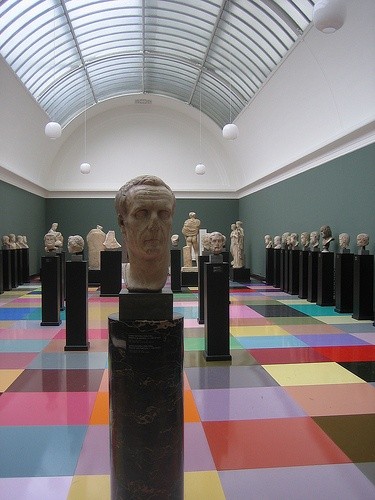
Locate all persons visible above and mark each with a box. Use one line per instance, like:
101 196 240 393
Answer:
338 232 350 249
200 233 212 251
357 233 369 247
232 220 244 269
182 212 200 267
310 231 320 247
43 223 64 252
0 233 30 249
301 232 310 247
208 232 227 252
67 235 84 254
114 175 174 292
320 225 335 246
229 224 238 266
264 231 299 250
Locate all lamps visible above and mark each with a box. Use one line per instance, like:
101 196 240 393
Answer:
313 0 345 34
222 50 240 140
45 0 62 140
80 65 91 174
196 75 206 175
